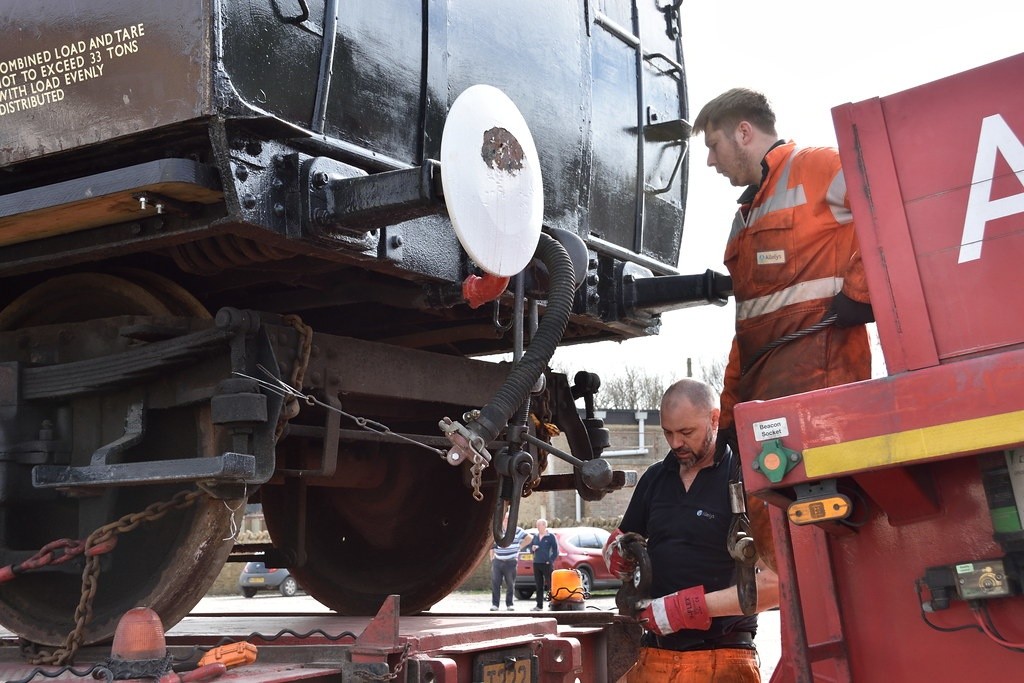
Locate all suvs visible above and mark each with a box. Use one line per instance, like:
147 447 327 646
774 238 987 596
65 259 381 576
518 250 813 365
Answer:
512 526 625 603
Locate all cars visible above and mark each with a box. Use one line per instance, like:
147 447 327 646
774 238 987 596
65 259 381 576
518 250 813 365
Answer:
239 548 304 599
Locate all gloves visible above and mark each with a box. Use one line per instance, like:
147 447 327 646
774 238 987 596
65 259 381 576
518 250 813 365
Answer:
714 426 741 467
600 528 649 582
822 291 875 325
632 585 712 636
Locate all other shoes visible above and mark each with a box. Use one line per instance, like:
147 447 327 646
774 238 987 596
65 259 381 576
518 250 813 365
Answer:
530 606 544 611
490 605 499 611
506 605 514 611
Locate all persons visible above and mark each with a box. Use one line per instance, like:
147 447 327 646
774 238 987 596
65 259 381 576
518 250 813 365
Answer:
601 378 780 683
691 88 876 574
490 512 559 611
